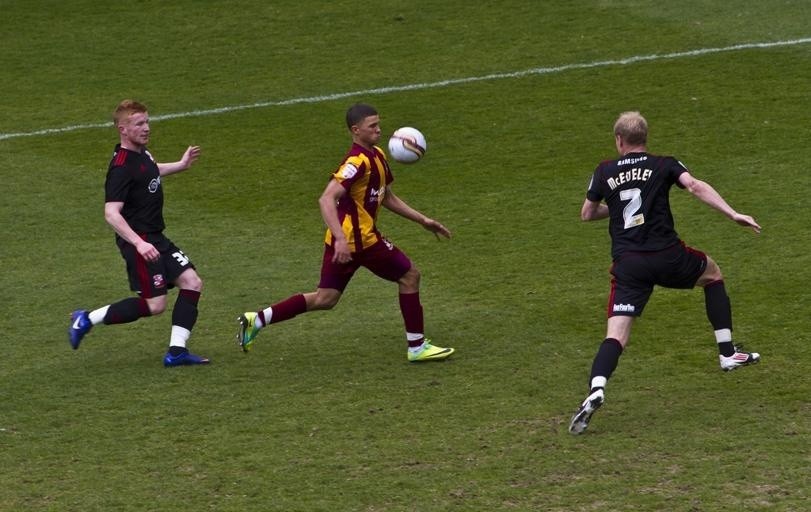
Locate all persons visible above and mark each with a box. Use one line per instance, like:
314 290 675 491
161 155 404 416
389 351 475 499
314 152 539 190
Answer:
69 98 210 367
235 103 455 363
569 111 762 438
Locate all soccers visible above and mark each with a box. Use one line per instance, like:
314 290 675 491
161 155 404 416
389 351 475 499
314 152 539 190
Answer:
388 127 425 164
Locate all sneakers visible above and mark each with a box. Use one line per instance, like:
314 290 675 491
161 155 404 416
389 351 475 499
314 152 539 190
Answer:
569 387 604 435
407 338 455 362
164 349 211 367
236 312 260 353
719 346 761 373
68 309 92 350
